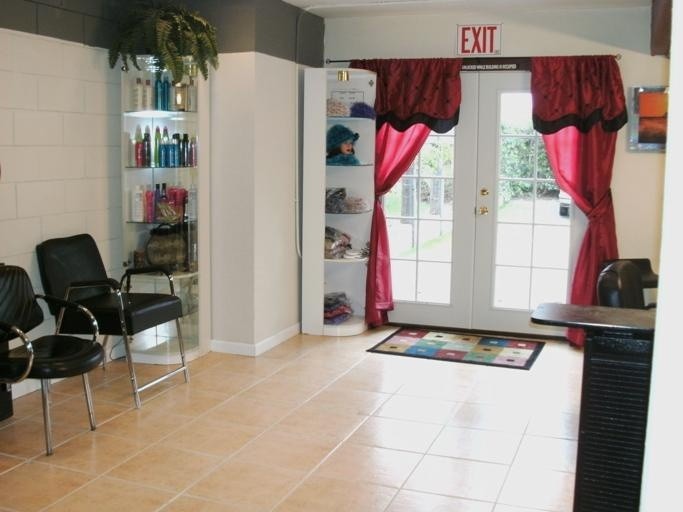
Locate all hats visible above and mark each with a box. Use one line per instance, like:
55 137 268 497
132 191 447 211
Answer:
326 188 370 213
326 126 358 149
325 99 349 118
351 102 376 117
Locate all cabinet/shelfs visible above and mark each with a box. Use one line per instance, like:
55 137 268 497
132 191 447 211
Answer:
302 67 376 336
121 55 212 365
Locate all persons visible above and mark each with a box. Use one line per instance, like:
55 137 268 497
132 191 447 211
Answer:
326 123 360 157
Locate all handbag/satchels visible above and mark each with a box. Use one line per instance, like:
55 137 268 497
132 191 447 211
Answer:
145 224 186 265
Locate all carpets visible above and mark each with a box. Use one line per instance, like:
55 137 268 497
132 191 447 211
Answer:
366 327 546 371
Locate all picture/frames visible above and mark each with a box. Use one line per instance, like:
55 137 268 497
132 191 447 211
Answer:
627 85 670 152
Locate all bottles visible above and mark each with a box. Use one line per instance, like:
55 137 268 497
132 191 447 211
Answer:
131 182 196 222
129 124 197 168
132 74 197 111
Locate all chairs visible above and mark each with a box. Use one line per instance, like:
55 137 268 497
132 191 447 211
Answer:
596 259 658 311
0 265 105 456
36 231 189 407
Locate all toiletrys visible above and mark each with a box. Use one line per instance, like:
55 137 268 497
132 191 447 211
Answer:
159 137 180 168
135 76 169 111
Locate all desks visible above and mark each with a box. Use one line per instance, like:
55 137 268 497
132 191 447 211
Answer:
531 302 655 512
